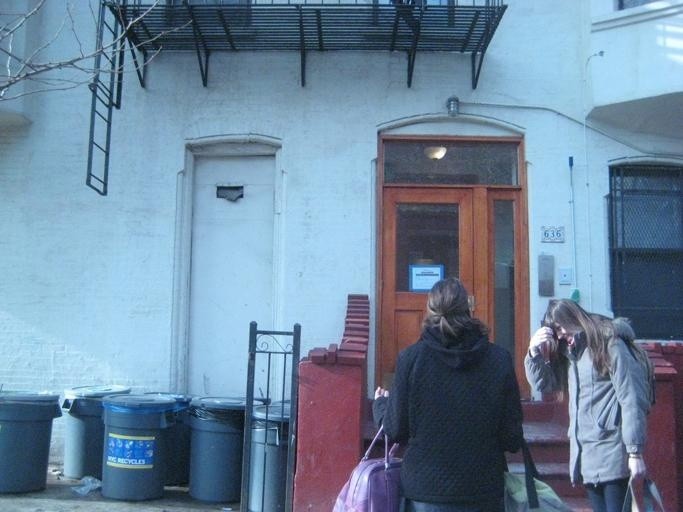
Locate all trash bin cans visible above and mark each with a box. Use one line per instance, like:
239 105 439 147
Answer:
184 396 270 504
165 392 191 487
62 385 131 479
1 391 62 493
101 394 178 501
247 405 290 512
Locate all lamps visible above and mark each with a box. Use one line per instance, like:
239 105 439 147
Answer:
446 94 460 111
422 145 447 162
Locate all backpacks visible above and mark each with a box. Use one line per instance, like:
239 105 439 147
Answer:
622 335 655 415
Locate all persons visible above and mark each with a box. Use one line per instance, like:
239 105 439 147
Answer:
372 279 523 512
524 299 656 512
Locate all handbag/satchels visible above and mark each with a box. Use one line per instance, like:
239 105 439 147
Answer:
331 457 403 512
504 475 573 512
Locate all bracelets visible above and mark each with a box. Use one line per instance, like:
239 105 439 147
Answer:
629 456 641 459
